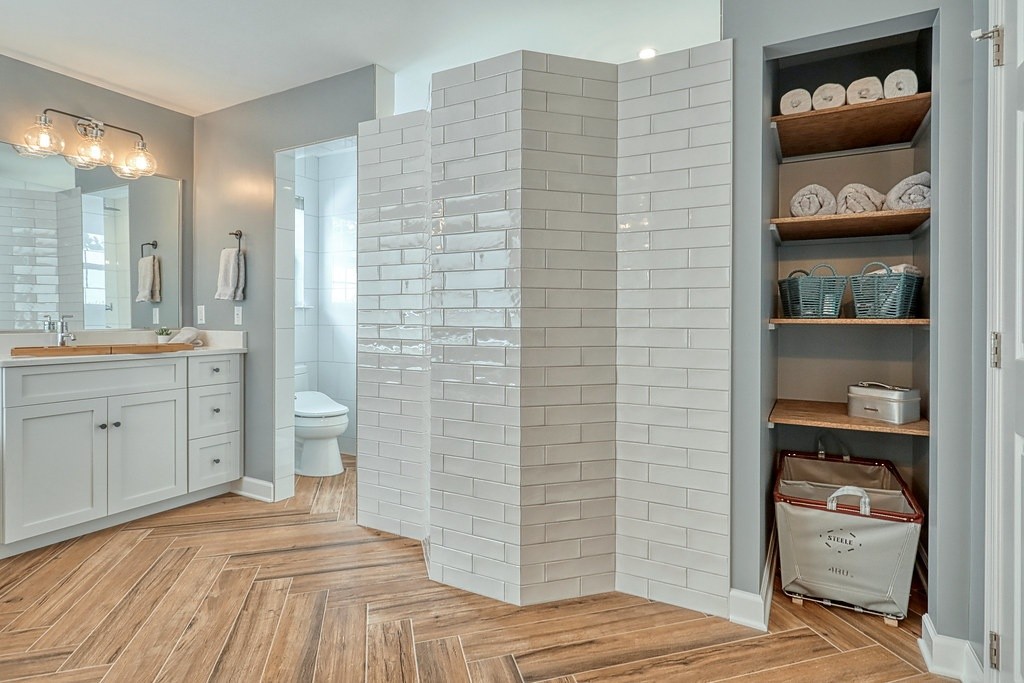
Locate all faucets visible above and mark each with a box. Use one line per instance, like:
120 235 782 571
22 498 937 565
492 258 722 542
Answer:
43 315 55 333
57 315 77 347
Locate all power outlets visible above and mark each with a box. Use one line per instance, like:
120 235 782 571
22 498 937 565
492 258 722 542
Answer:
153 308 159 325
234 306 243 326
197 305 205 325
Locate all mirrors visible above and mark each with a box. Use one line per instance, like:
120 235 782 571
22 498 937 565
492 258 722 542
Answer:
0 141 184 335
294 195 304 309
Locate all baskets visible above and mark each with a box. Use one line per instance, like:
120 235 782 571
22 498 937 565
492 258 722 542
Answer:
777 264 849 318
850 262 924 318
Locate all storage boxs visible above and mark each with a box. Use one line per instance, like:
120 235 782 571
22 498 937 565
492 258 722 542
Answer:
773 448 925 628
847 381 921 425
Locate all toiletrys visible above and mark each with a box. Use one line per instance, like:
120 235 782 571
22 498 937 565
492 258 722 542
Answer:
822 280 838 316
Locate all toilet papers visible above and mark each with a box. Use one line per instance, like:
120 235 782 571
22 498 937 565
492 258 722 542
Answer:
780 69 919 116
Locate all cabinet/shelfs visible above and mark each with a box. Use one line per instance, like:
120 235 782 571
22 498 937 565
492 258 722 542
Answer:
0 353 245 559
767 90 932 444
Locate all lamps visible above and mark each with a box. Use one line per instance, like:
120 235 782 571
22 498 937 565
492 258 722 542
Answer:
12 144 142 180
25 108 158 177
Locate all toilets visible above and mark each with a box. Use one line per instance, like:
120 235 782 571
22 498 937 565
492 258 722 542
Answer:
294 365 350 477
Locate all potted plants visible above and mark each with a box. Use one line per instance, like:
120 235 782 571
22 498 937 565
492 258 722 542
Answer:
154 327 172 345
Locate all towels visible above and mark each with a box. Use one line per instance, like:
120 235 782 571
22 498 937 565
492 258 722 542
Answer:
135 255 161 305
213 249 246 302
168 327 203 347
789 171 932 217
856 263 922 317
